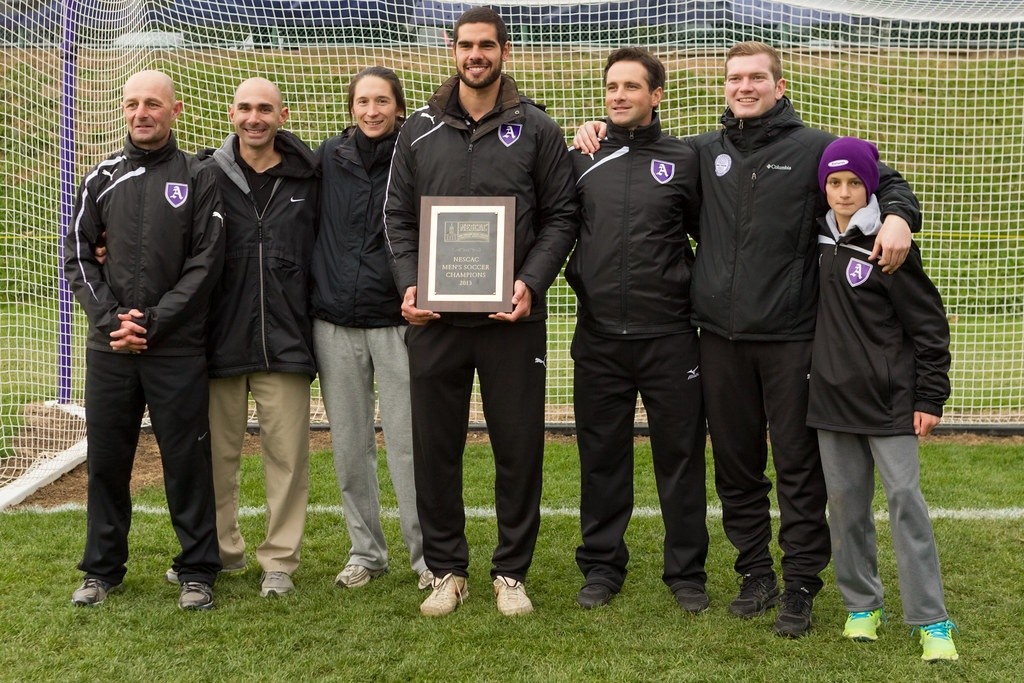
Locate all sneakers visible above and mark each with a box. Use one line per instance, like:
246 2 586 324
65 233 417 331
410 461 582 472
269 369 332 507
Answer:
728 569 777 617
673 586 710 613
177 582 214 611
166 565 251 584
842 609 883 641
492 575 533 617
261 572 295 597
421 575 470 617
419 569 434 590
335 565 385 589
579 585 613 609
919 621 961 663
72 579 123 607
773 590 815 640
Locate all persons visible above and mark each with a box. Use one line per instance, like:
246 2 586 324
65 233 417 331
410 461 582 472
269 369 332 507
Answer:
314 66 436 590
93 77 322 597
806 138 959 662
381 8 579 617
572 42 925 639
63 69 225 613
556 47 708 610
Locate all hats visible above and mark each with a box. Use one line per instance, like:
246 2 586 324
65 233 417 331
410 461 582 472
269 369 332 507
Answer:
817 137 880 202
